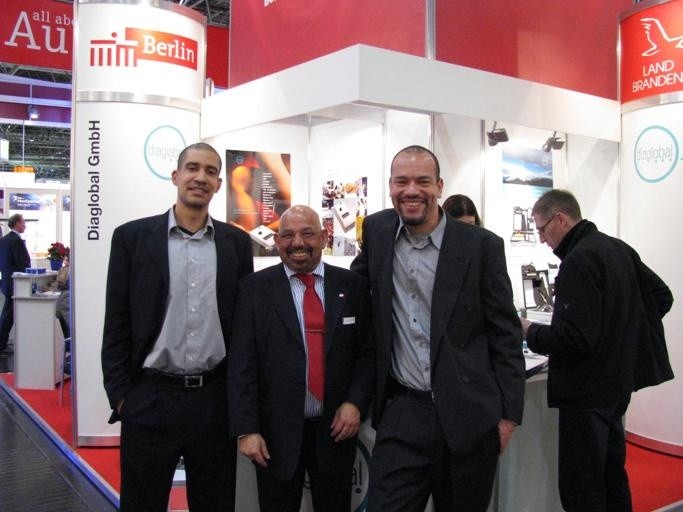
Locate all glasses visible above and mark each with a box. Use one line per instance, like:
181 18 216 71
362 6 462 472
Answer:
535 212 559 234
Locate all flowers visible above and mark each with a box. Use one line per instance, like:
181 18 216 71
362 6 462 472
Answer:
48 242 65 261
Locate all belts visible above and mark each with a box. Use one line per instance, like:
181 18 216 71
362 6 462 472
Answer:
142 366 228 389
392 379 435 403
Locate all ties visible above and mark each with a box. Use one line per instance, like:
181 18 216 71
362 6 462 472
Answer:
295 273 328 401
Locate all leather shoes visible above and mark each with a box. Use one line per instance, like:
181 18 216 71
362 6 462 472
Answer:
0 347 14 355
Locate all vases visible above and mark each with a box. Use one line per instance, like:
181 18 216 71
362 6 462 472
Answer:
49 255 62 270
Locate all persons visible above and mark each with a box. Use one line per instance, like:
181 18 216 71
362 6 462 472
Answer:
439 194 482 229
512 189 678 509
0 213 32 357
222 203 380 511
38 252 71 366
98 140 255 512
227 151 293 237
345 143 526 511
